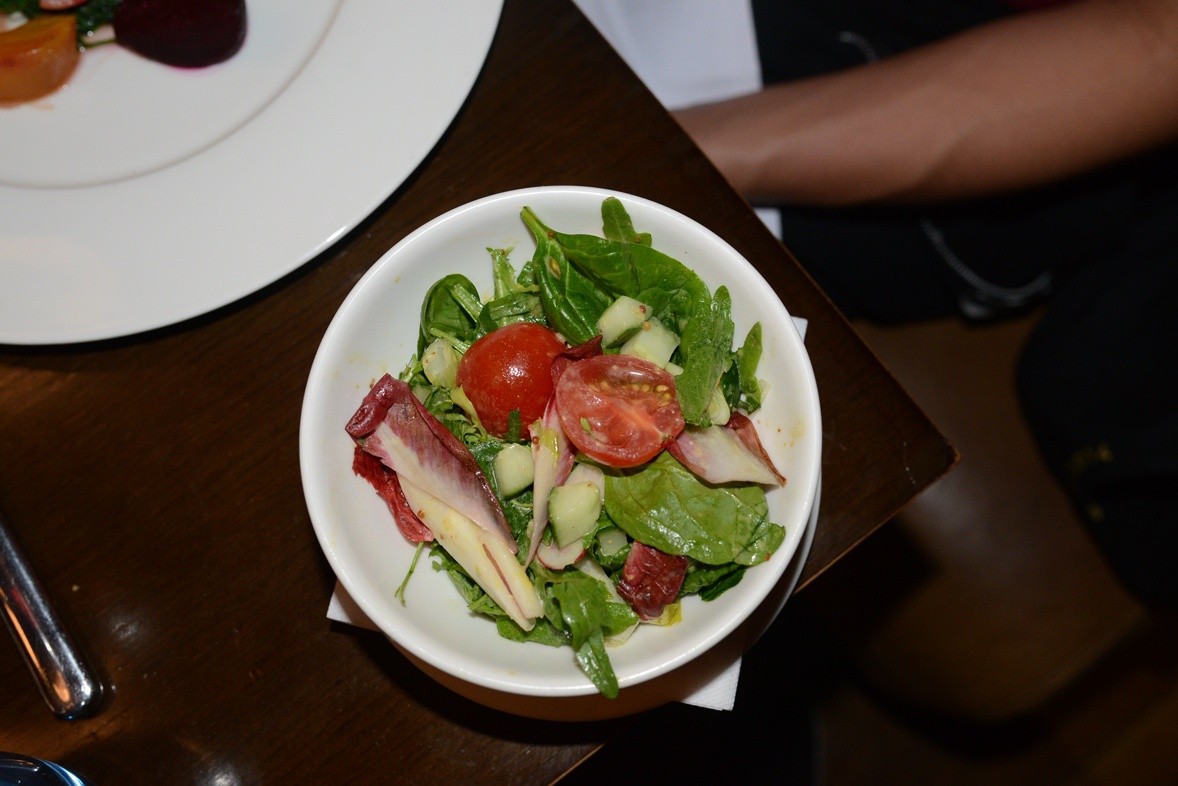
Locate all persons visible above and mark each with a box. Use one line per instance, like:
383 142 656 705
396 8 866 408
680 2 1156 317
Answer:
667 1 1178 306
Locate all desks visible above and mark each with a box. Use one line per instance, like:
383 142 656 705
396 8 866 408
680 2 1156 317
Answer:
0 0 963 786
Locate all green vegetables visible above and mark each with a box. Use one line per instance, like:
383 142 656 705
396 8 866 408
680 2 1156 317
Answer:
0 0 118 47
341 197 785 701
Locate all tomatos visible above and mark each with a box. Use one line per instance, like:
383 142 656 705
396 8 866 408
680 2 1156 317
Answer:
455 321 569 442
554 354 687 467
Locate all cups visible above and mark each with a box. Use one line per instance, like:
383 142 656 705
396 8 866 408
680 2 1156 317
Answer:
0 749 86 786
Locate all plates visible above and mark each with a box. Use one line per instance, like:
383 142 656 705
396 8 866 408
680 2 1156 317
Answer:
296 184 825 697
0 0 506 349
388 468 820 724
